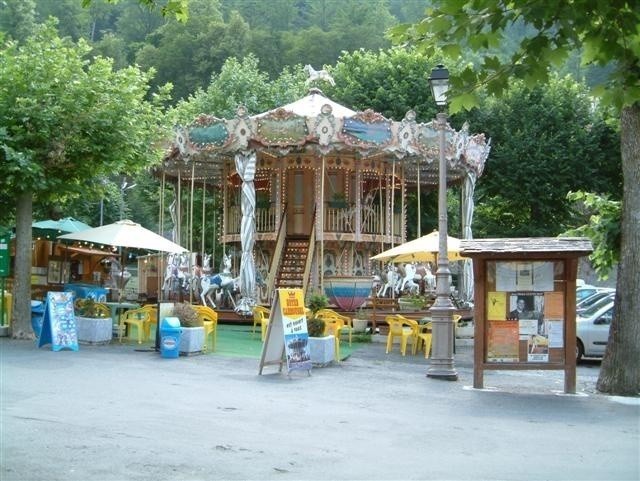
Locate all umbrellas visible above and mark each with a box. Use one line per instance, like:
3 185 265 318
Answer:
368 229 471 290
55 219 190 325
12 217 94 284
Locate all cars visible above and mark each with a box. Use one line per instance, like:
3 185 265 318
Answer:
576 279 618 362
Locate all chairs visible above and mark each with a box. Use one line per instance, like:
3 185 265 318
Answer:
384 314 461 360
252 307 351 360
119 304 217 355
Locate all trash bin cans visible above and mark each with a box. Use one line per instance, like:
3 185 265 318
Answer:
30 300 45 336
160 317 182 358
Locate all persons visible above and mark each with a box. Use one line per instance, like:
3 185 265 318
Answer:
508 297 532 320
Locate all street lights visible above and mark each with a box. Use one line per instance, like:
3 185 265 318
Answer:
119 181 137 220
425 62 461 381
